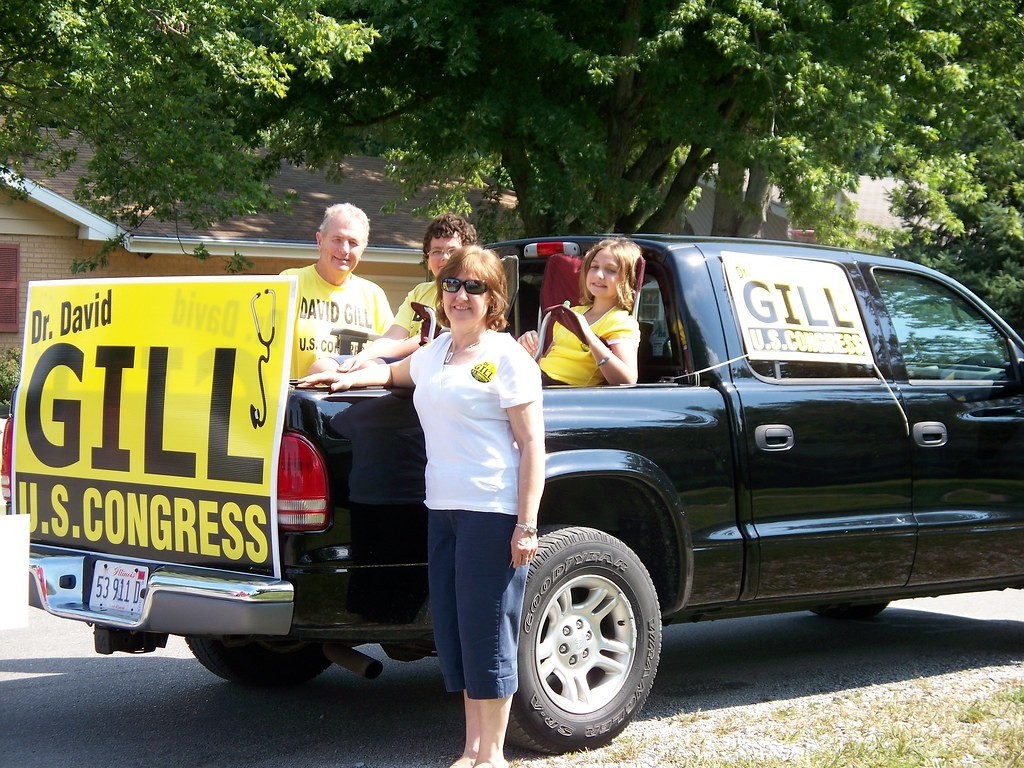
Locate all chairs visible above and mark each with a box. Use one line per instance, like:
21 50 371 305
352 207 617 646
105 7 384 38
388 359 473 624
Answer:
331 255 521 361
538 252 645 328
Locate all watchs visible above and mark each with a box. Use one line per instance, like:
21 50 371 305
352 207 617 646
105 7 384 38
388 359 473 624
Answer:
516 522 537 533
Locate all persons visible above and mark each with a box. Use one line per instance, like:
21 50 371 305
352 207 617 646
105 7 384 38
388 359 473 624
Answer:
516 234 645 388
295 246 546 768
282 201 395 378
344 211 478 389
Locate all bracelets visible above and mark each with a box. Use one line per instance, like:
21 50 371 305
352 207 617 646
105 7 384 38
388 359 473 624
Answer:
596 350 613 365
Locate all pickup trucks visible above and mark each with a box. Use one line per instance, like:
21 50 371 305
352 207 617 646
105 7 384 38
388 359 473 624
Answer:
2 231 1022 757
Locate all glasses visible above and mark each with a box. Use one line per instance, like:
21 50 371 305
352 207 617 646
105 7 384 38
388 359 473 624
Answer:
441 278 487 294
427 248 455 256
